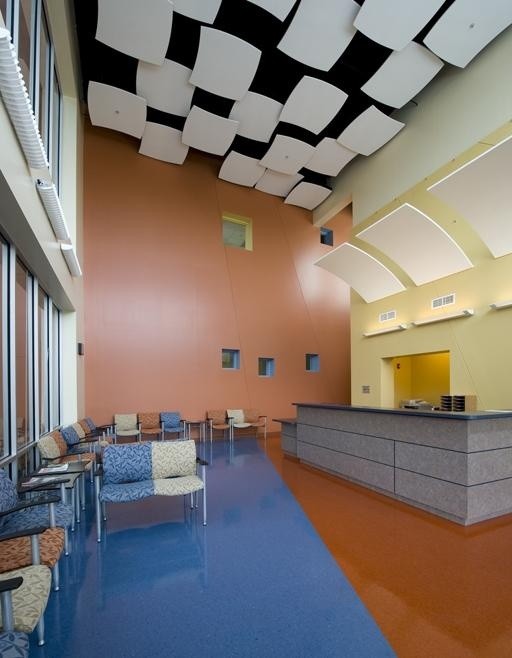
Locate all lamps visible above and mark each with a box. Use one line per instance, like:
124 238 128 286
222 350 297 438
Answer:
62 243 82 277
490 300 512 310
0 28 49 169
363 324 407 336
412 309 473 327
36 180 70 240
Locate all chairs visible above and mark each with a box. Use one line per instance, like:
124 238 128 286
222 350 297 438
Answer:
1 411 111 656
96 440 208 533
114 408 266 442
